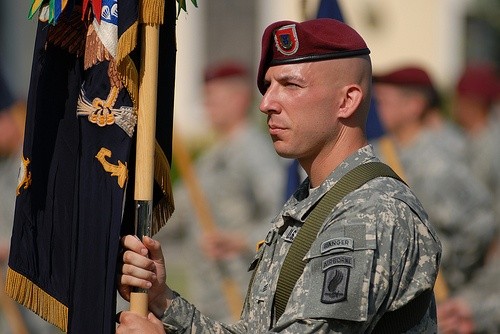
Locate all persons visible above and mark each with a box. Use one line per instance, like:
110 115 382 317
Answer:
153 58 291 322
0 75 61 334
371 58 500 334
115 18 442 334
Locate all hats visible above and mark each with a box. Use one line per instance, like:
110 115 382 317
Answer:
457 65 500 99
204 63 250 81
373 68 431 85
257 19 371 96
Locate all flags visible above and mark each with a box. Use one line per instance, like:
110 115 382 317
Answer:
282 0 386 208
6 0 191 332
